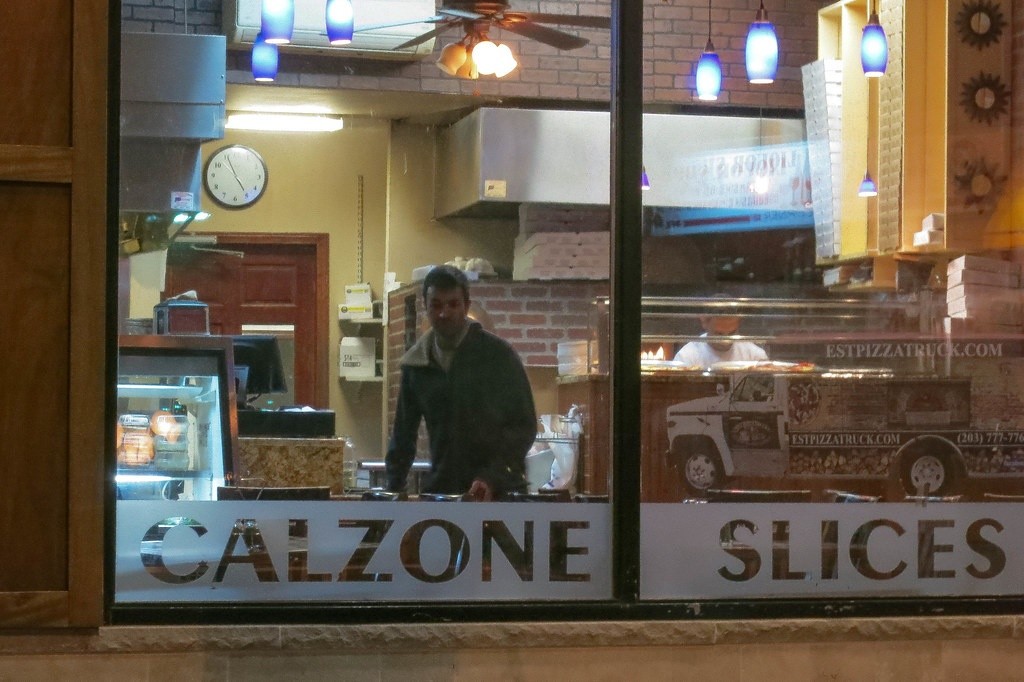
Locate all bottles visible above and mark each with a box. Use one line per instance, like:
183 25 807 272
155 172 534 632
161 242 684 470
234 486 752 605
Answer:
342 435 357 488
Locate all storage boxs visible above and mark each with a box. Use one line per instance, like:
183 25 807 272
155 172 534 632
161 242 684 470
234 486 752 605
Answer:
946 254 1020 319
338 336 376 378
338 284 373 319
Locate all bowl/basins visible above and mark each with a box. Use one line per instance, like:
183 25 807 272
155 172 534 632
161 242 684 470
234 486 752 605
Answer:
124 317 153 334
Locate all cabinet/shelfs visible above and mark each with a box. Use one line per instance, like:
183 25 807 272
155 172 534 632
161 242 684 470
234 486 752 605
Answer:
347 317 383 402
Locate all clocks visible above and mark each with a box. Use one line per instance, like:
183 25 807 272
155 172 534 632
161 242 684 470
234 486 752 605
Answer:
204 145 268 209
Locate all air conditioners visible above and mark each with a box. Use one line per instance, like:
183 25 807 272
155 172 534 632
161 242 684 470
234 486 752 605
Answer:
221 0 436 63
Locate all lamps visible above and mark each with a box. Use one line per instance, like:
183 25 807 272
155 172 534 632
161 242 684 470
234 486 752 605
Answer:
861 0 888 78
325 0 353 45
261 0 295 44
251 32 281 82
744 0 780 84
858 137 878 196
693 0 722 101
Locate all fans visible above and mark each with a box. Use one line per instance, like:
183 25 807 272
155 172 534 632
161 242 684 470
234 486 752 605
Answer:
353 0 611 79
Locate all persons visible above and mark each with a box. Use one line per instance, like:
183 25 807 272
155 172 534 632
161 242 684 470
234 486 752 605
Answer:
385 265 537 501
673 294 768 361
